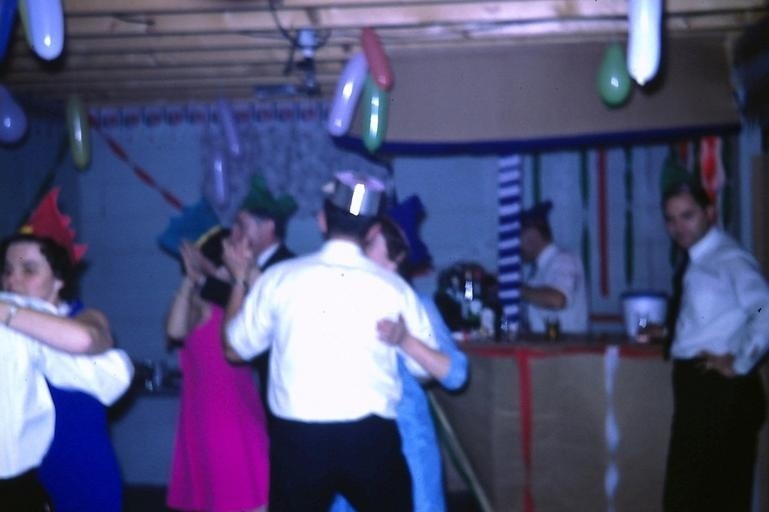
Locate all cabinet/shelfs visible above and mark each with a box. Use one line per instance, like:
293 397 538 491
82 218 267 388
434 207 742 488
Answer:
422 329 674 511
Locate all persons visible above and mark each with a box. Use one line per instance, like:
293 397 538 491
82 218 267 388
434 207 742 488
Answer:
222 168 440 511
1 292 136 509
329 192 471 512
636 162 769 510
1 187 124 510
488 199 589 337
176 184 303 305
165 196 272 512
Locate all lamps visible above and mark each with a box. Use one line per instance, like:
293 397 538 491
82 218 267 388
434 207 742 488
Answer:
276 26 323 100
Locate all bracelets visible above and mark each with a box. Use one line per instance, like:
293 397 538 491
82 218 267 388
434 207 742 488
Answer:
661 325 668 339
178 287 193 304
232 277 250 291
3 303 20 328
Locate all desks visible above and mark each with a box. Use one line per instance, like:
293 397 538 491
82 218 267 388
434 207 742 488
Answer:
108 375 183 487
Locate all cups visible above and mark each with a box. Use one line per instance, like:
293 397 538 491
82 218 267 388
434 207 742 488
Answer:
545 316 558 341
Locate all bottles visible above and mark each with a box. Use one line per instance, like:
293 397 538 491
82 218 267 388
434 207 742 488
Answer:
451 265 486 330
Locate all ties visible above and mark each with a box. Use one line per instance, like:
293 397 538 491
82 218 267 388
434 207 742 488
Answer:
662 249 688 360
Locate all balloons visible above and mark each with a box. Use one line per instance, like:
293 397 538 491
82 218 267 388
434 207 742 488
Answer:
324 53 363 136
1 1 72 61
1 84 29 144
363 76 389 151
212 148 229 206
627 0 663 84
65 92 93 169
363 27 392 86
596 43 629 104
221 99 244 158
